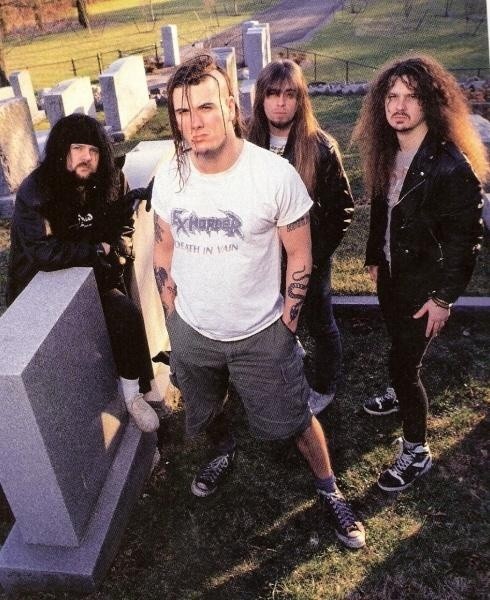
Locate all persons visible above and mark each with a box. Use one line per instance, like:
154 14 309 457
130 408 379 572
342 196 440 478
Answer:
245 57 356 417
4 114 163 434
150 55 367 551
342 53 488 493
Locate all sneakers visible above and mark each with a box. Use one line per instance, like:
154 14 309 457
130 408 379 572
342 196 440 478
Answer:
192 453 231 496
126 394 159 433
363 389 399 414
309 388 334 416
318 488 365 549
377 441 433 490
